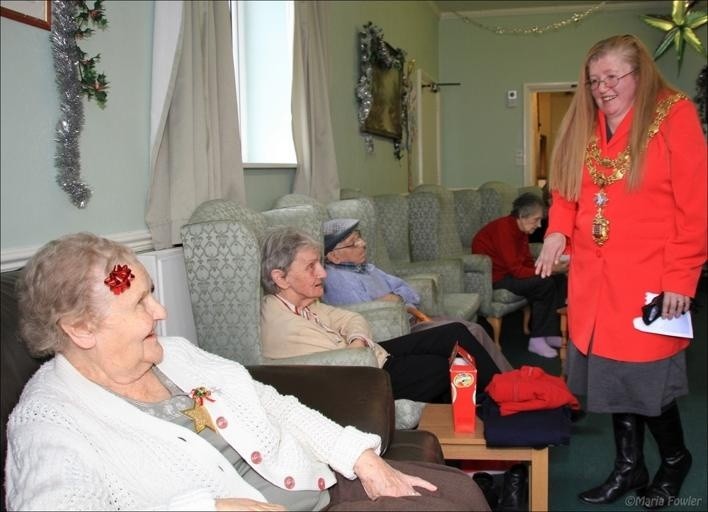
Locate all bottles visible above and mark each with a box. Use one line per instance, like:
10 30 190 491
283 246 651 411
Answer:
323 218 361 256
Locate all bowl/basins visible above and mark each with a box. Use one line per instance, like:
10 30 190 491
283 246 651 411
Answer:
577 94 692 245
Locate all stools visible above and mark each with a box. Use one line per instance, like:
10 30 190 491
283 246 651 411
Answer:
414 402 549 511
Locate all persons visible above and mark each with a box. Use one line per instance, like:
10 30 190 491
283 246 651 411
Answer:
528 33 707 510
257 226 506 410
311 218 520 378
465 192 570 361
0 230 498 512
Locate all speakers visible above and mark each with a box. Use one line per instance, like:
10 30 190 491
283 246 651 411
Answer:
577 411 650 506
643 397 694 511
472 463 529 511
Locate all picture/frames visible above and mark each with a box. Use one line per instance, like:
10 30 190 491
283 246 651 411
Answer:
362 25 406 140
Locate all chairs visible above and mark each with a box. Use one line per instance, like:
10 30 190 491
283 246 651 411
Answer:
1 179 544 512
528 336 562 359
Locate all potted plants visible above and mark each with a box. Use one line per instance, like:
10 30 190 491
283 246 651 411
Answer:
362 25 406 140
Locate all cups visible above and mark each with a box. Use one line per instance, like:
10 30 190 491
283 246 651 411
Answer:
333 230 360 251
584 67 640 91
